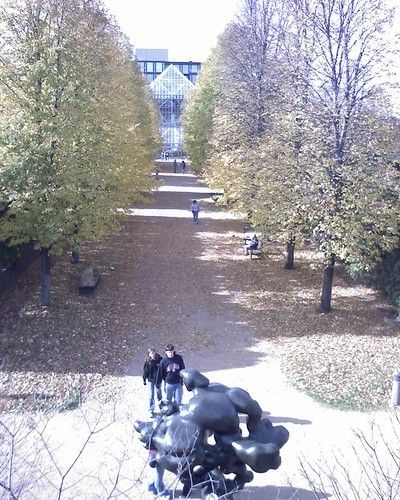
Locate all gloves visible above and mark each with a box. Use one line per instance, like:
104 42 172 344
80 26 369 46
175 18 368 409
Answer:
143 382 146 386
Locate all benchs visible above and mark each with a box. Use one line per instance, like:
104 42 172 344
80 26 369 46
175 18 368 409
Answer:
243 238 263 259
211 192 229 205
79 268 101 297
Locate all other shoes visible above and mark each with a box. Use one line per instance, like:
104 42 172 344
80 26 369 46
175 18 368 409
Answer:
150 412 154 418
160 407 164 413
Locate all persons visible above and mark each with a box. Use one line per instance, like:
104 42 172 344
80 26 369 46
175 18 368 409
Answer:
180 160 185 174
157 344 185 408
143 348 164 419
244 233 259 255
173 159 177 173
192 200 199 224
